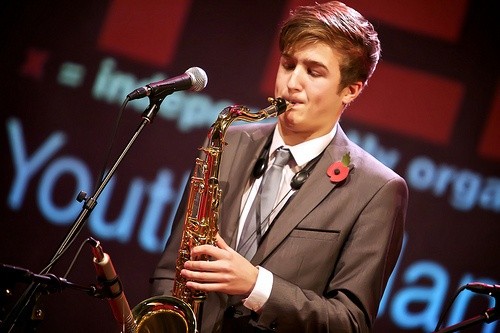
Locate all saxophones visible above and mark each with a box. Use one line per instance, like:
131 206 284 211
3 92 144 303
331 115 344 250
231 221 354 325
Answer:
122 96 294 333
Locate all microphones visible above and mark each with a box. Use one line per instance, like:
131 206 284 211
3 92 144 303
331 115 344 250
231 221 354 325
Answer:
466 282 500 298
127 67 208 101
91 241 138 333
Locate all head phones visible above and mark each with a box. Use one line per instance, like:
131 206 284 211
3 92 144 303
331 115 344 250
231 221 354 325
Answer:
252 127 330 190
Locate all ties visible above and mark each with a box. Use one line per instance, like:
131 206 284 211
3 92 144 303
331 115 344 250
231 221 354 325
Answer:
235 145 291 262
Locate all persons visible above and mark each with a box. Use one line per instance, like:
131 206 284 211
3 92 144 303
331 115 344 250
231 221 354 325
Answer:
151 0 409 333
